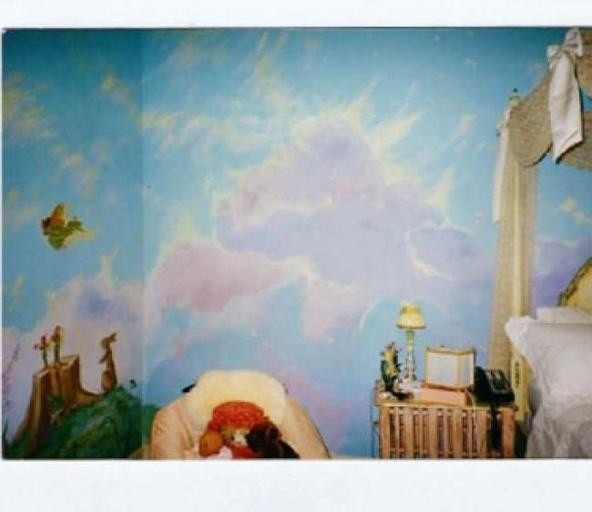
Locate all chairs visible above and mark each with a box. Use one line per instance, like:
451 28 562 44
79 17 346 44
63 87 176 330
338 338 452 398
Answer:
151 368 333 459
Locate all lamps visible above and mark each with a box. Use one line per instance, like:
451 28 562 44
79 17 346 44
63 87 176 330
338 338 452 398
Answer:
395 305 427 381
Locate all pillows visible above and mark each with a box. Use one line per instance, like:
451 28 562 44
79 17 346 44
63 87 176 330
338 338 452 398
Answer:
504 306 592 422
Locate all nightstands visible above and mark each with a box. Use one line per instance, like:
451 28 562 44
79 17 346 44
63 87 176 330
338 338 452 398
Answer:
375 379 519 458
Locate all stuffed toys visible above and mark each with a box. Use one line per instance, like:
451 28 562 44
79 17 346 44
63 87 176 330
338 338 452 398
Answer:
198 400 301 459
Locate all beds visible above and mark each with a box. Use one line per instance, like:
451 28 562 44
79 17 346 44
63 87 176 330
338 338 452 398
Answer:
489 29 592 458
